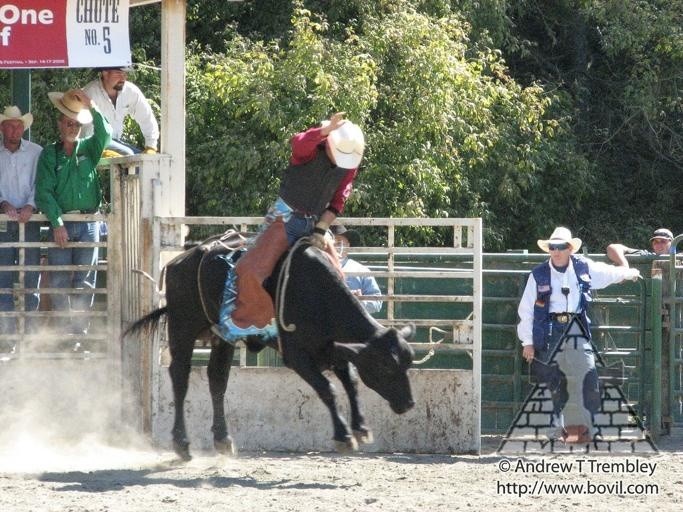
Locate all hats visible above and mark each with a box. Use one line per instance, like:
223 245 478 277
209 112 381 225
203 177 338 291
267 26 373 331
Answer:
320 119 364 169
92 66 135 71
537 227 581 253
0 105 33 131
329 225 359 243
648 228 673 241
47 91 93 124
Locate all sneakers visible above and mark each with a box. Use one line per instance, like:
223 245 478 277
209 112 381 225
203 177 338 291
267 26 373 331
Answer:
547 426 567 445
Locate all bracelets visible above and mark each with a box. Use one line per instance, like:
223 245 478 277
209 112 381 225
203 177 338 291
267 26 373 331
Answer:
311 222 328 237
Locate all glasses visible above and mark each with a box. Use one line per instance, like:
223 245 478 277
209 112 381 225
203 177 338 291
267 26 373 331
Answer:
67 121 82 127
548 243 569 250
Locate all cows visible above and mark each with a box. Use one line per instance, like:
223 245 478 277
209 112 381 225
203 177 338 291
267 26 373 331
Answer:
121 231 416 462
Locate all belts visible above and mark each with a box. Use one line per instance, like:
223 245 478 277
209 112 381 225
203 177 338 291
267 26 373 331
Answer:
549 314 571 323
294 211 312 219
65 210 91 214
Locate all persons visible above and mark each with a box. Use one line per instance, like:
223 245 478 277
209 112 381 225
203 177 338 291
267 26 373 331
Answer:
31 89 113 360
329 223 384 316
209 112 367 348
606 228 674 284
0 104 44 361
78 66 161 176
515 226 644 448
